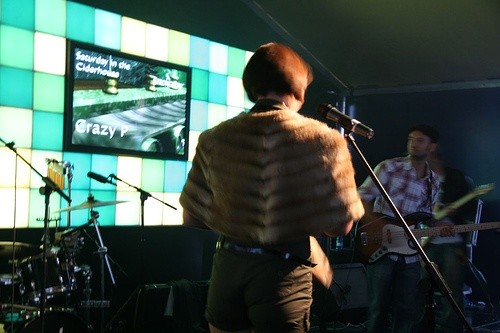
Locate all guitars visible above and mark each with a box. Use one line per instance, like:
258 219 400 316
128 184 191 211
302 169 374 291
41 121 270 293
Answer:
356 209 487 265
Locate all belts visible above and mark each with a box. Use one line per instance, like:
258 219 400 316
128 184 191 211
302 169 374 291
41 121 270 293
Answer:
215 241 317 267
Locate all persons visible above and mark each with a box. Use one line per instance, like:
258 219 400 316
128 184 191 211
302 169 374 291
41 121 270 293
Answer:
356 125 478 333
179 42 365 333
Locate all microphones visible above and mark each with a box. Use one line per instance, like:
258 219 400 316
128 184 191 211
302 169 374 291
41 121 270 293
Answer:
88 170 117 189
315 103 387 139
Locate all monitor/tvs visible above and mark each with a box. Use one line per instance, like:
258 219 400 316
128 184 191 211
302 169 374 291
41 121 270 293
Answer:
62 38 192 162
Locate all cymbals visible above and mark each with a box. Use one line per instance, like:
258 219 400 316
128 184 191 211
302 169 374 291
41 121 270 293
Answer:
54 199 127 211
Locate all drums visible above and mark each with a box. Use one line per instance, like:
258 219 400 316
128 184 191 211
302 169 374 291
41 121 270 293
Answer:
20 251 79 304
